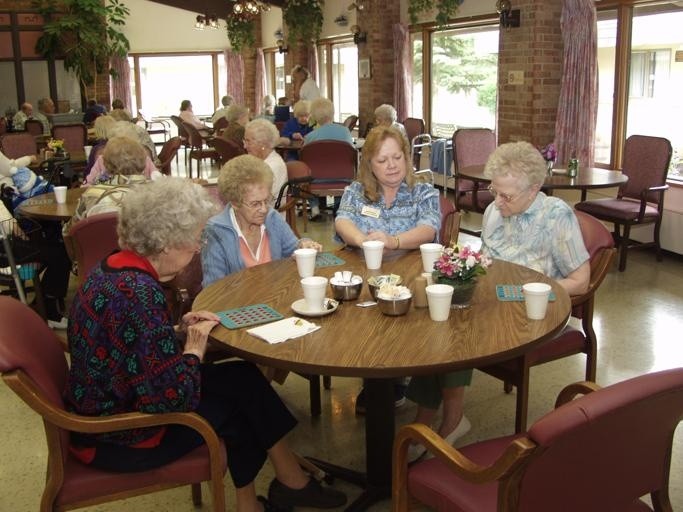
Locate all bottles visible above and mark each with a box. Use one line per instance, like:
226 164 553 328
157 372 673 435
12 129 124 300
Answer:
412 272 435 309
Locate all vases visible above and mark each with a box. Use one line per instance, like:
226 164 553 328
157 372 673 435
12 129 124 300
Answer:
546 161 553 172
435 273 476 312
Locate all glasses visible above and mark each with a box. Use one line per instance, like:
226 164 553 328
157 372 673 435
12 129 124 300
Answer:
486 184 532 202
241 195 275 208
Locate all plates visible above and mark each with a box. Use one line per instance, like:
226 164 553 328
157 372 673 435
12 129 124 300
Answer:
290 297 338 318
551 168 567 174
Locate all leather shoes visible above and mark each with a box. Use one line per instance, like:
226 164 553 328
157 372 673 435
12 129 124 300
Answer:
404 412 471 463
268 477 347 512
48 315 68 329
308 214 321 221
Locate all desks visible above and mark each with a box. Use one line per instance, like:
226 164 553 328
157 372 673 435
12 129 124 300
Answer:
192 246 573 511
458 167 630 209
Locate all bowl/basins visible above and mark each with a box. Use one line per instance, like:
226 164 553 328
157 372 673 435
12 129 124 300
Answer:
369 275 403 301
329 282 363 302
366 283 412 318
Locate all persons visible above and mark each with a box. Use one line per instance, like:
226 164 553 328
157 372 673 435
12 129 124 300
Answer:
290 66 320 100
200 153 324 380
0 150 72 327
12 96 55 136
374 103 410 154
62 99 160 237
332 121 444 409
181 95 354 220
60 176 347 512
404 139 591 463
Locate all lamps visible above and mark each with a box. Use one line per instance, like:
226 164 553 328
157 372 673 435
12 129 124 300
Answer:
233 0 272 16
195 10 220 31
350 26 367 44
277 40 288 54
496 0 520 33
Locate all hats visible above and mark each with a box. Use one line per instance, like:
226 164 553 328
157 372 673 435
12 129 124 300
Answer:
9 167 37 192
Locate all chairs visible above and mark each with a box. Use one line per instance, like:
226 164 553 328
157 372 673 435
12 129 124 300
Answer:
471 210 615 433
451 127 497 214
574 134 674 273
390 366 682 511
1 294 230 511
1 116 451 294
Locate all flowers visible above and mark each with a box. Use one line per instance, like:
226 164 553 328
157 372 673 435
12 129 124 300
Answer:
542 144 559 160
433 247 490 273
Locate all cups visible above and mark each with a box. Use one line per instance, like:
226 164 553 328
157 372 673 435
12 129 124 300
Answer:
52 185 67 204
419 242 442 274
424 283 455 322
293 247 318 278
300 275 330 311
521 281 551 321
361 239 384 271
83 145 93 159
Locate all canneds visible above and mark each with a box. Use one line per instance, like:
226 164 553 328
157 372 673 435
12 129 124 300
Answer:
566 159 578 178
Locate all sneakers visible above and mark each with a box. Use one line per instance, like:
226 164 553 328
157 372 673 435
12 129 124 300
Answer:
354 385 406 411
